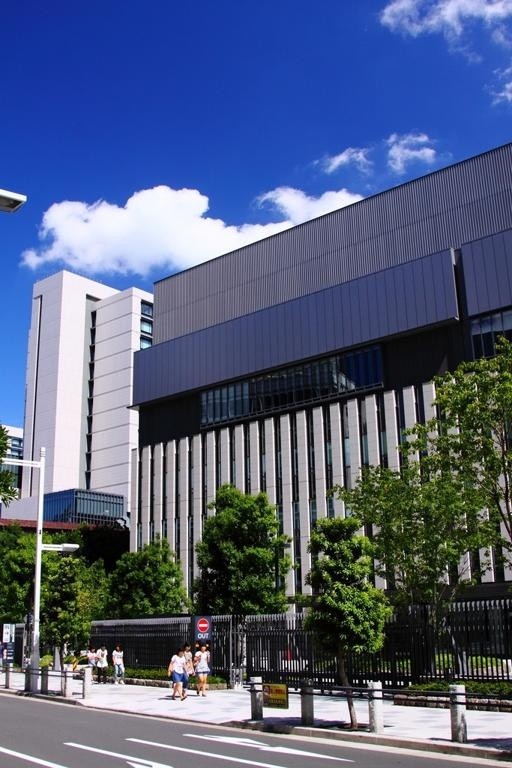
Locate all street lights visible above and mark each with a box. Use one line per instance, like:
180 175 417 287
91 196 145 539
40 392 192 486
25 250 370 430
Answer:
25 447 80 692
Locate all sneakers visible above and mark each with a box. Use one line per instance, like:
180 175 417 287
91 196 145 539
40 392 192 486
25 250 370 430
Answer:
98 679 106 684
172 695 186 701
196 687 206 696
115 679 124 684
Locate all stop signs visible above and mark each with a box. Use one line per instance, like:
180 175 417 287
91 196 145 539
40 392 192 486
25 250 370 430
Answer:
193 616 212 641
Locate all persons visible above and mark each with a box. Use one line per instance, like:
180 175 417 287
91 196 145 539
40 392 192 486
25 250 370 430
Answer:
96 641 109 684
167 646 190 701
194 640 211 696
111 643 125 685
87 646 103 673
172 641 196 697
